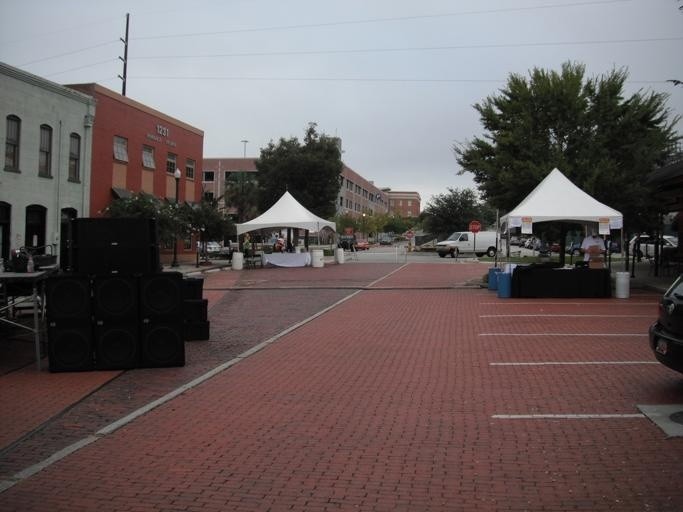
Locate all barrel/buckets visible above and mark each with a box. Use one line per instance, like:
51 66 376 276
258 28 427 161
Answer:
496 272 512 298
232 252 243 270
335 248 344 264
616 272 630 298
488 269 500 290
311 249 323 267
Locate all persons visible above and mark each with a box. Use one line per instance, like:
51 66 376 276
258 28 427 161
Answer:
243 232 250 243
350 242 358 262
579 234 607 267
271 234 278 244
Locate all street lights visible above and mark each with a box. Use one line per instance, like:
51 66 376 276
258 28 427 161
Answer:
171 168 181 267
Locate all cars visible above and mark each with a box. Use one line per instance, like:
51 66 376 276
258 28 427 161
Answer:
629 234 679 258
358 242 371 250
200 242 221 253
649 272 683 374
510 236 580 255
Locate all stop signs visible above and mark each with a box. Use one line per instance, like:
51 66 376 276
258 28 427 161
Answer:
469 221 481 232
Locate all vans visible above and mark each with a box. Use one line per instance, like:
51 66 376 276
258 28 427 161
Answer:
436 232 501 258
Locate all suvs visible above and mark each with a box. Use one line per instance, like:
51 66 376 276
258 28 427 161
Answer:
338 234 358 252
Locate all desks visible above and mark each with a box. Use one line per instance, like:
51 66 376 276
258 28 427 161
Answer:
512 265 611 298
0 269 49 372
262 251 312 268
